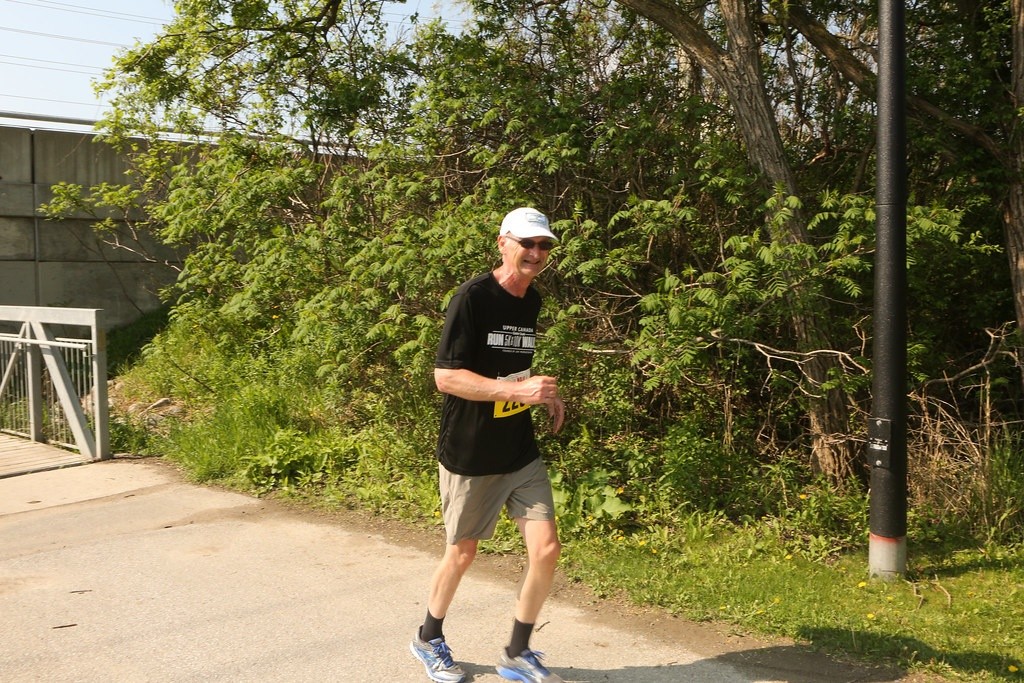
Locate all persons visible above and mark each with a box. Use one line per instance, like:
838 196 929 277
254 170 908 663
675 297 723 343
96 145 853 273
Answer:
410 207 565 683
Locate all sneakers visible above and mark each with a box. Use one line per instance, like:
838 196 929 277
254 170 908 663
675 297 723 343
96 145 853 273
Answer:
410 624 467 683
495 643 564 683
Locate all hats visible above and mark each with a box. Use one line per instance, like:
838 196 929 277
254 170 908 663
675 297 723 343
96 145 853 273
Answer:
501 207 559 243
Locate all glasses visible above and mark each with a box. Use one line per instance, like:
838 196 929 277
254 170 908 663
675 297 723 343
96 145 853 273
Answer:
504 235 554 250
